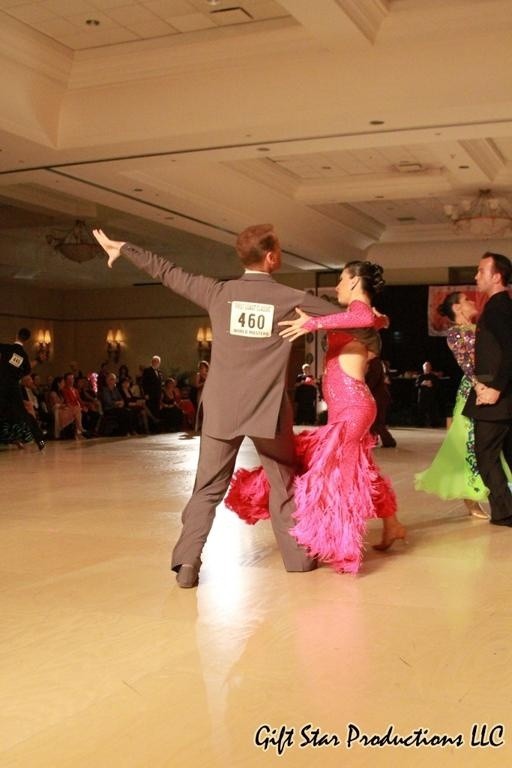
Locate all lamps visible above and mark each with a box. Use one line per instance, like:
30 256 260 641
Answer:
437 184 511 241
105 327 127 364
195 324 212 363
49 217 107 264
33 328 53 358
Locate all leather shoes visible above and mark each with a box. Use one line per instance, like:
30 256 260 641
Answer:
380 442 397 448
489 513 511 528
37 440 47 451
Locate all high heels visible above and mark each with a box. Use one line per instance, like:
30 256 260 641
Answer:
464 499 491 520
373 524 409 553
74 427 87 442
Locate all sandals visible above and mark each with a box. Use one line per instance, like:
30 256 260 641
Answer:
15 440 25 450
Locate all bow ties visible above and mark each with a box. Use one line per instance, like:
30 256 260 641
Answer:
153 368 159 372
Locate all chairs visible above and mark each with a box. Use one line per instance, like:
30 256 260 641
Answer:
41 388 182 440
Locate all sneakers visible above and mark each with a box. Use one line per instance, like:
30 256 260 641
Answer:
175 563 199 590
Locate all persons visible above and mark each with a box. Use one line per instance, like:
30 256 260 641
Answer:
92 224 384 589
461 252 512 530
222 259 408 575
413 291 512 518
0 328 446 448
